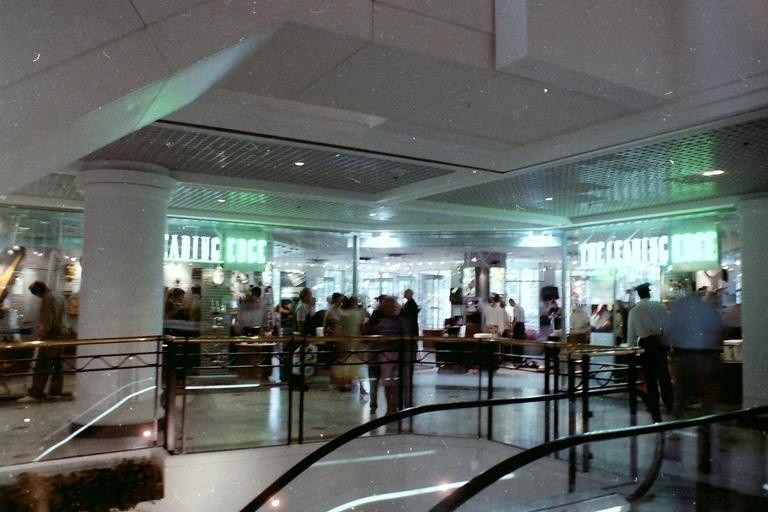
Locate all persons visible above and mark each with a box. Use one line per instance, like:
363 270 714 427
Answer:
293 287 312 336
546 299 561 330
323 293 344 371
499 301 509 362
660 278 730 482
334 297 366 392
483 293 505 363
16 279 65 405
713 268 737 309
480 296 493 333
403 289 419 361
508 298 525 365
162 286 201 375
367 295 410 414
234 287 264 335
623 282 673 423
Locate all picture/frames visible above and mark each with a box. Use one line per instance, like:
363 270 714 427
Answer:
423 328 504 368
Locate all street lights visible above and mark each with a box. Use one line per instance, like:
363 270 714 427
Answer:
290 306 311 326
292 344 319 376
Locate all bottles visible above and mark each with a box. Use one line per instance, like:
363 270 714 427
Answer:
635 282 653 293
